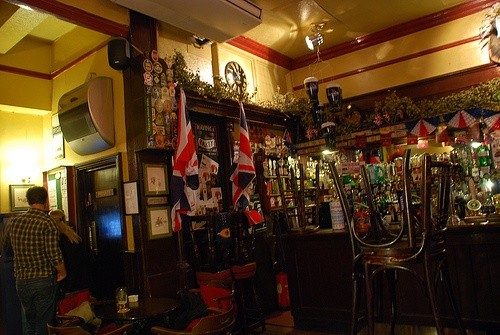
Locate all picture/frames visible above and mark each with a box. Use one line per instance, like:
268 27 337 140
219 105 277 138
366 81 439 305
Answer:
144 163 172 241
211 42 258 105
10 184 36 212
122 180 140 215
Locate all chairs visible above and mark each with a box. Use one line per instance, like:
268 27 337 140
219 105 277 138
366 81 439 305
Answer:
47 260 238 335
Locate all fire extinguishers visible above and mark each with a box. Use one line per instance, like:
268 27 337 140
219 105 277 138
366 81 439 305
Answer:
276 271 290 309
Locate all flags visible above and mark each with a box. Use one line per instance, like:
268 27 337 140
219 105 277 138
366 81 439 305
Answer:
168 87 200 232
228 101 257 211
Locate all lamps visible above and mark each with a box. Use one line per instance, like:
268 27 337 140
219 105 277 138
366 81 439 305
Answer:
305 23 324 50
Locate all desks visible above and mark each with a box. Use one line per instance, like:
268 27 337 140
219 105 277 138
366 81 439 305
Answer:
94 297 180 335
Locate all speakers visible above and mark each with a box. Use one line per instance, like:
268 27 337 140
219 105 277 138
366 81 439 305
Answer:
107 37 130 70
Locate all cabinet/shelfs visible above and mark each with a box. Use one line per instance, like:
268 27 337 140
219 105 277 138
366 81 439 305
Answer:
279 220 500 335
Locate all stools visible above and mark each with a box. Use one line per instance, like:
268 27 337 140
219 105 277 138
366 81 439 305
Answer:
328 150 468 335
195 261 267 335
252 152 322 233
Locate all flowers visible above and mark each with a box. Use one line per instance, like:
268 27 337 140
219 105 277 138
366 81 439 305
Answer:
302 77 500 140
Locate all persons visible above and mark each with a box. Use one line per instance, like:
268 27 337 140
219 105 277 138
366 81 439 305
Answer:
48 209 89 326
0 186 67 335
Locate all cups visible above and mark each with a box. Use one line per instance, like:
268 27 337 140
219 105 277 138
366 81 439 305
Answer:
115 288 130 313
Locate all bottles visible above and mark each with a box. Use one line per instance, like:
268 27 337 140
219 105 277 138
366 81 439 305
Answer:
264 158 292 194
383 204 392 226
307 157 318 188
